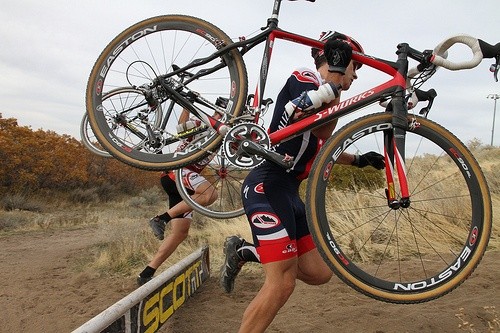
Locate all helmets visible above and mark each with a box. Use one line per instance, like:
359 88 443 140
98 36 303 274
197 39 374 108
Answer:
312 31 365 69
215 97 229 108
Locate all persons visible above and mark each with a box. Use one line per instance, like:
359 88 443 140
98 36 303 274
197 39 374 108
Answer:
220 30 385 333
136 97 229 285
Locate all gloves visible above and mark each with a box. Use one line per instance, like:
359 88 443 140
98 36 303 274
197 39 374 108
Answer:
350 151 386 170
323 39 353 74
186 90 200 102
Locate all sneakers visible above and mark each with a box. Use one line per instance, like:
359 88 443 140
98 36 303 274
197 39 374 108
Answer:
148 215 168 239
222 235 246 294
137 274 162 293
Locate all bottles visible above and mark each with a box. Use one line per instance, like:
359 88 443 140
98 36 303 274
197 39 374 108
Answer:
283 82 343 120
175 120 202 134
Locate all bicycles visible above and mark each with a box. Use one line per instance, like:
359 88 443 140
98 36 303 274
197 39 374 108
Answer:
79 60 247 219
84 0 500 305
